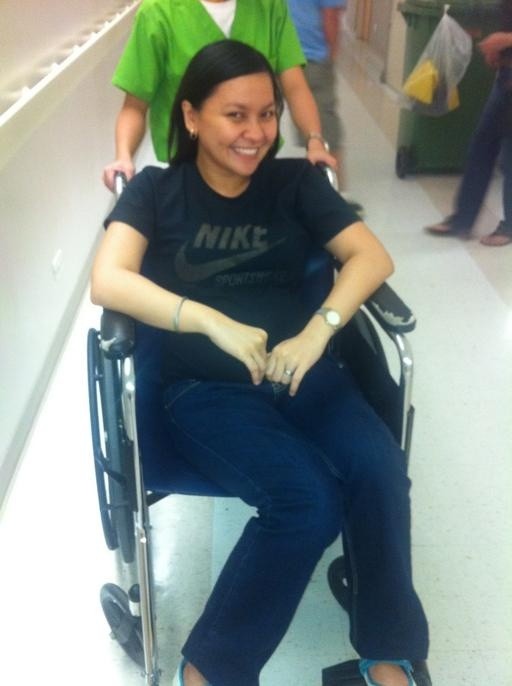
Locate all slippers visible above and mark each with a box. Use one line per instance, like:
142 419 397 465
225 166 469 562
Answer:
423 220 512 245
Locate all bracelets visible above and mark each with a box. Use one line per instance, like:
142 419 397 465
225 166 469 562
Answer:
173 295 192 334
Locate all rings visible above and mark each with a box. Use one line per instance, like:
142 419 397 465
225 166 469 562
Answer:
284 369 294 376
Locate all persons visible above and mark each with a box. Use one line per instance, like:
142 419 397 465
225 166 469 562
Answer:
101 1 339 198
278 1 349 194
91 37 433 686
420 1 511 247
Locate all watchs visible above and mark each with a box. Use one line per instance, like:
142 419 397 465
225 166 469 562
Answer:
305 132 329 154
312 307 344 332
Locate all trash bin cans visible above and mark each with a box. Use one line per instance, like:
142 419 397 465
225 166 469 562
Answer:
394 0 512 179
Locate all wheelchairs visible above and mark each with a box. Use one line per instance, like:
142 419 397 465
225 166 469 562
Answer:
86 142 430 686
